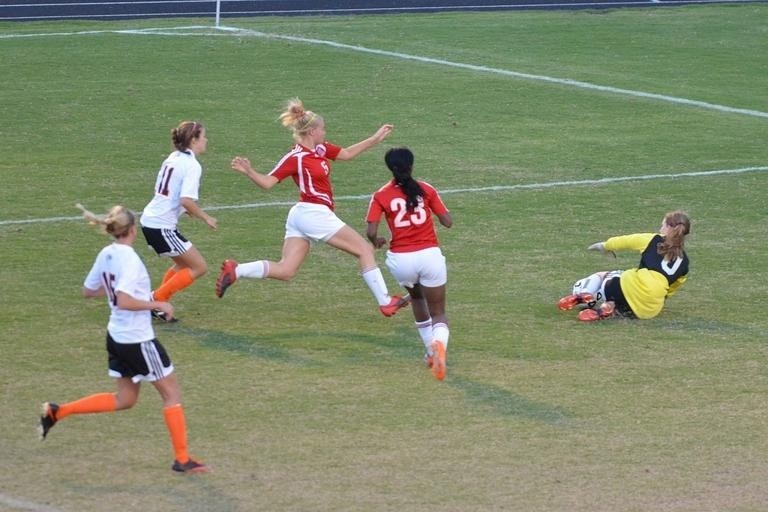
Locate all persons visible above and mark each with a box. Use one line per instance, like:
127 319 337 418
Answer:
35 204 210 477
557 211 691 322
364 146 453 382
138 121 219 322
215 96 413 318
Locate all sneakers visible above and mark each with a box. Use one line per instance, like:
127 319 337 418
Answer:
557 292 594 311
37 402 59 442
379 292 411 318
171 459 209 475
577 301 616 322
150 291 178 323
215 259 238 298
424 340 446 381
